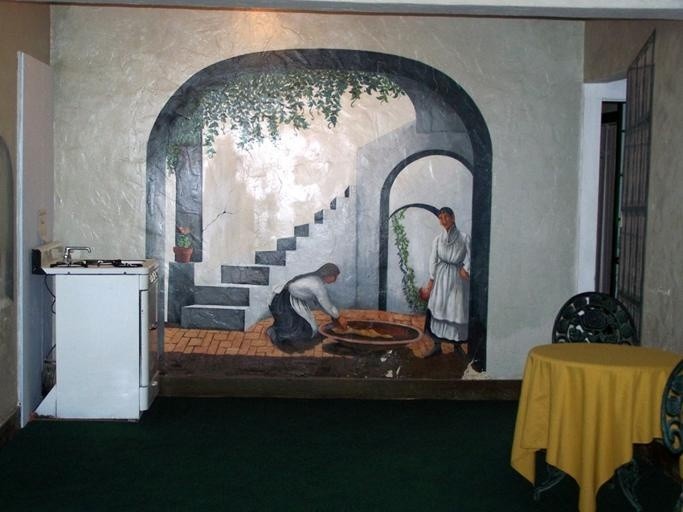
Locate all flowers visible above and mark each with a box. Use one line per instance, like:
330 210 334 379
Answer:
175 226 192 247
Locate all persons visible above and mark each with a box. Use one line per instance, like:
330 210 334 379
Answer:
266 262 348 354
423 205 470 354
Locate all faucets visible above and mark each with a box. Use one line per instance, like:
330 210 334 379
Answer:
62 245 91 263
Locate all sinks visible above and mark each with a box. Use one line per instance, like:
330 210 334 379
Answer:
49 259 147 267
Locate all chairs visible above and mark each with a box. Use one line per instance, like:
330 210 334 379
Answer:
528 292 683 512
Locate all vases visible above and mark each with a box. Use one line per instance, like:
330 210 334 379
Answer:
173 247 192 263
